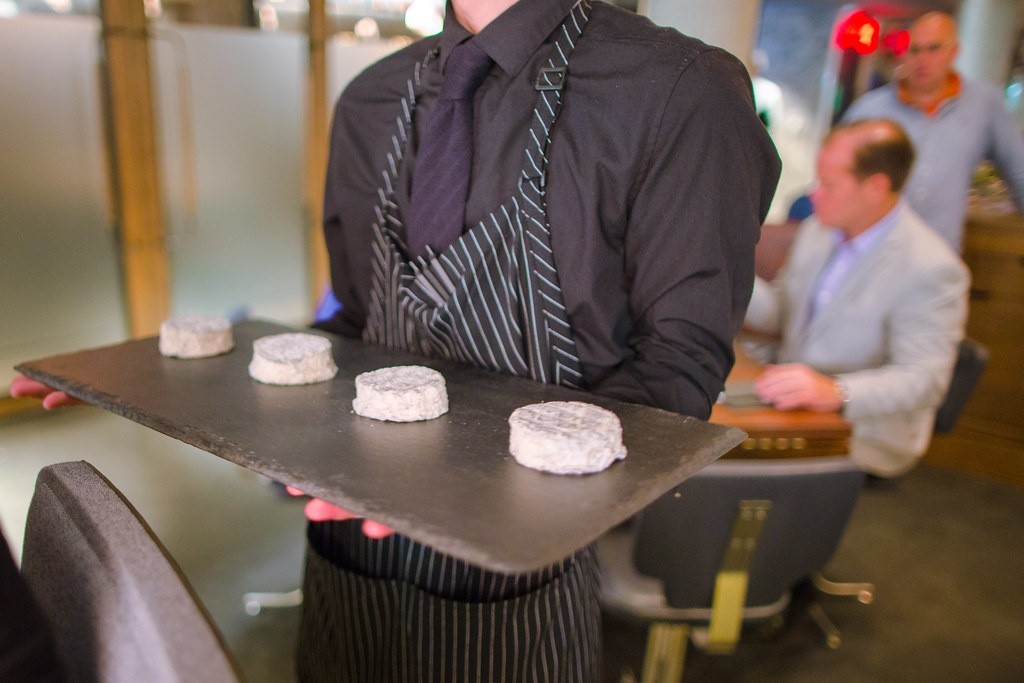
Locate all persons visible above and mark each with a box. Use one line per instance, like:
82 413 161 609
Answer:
753 117 969 490
837 11 1023 258
6 0 781 683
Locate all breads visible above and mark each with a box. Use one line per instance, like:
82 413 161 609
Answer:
352 364 448 422
158 312 235 359
507 400 628 474
249 332 339 385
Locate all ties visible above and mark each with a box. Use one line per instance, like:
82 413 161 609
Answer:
407 41 491 264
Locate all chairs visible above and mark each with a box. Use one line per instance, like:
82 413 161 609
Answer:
23 460 251 683
805 339 989 648
594 455 865 683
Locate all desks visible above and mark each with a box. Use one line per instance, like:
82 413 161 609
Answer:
704 343 852 456
